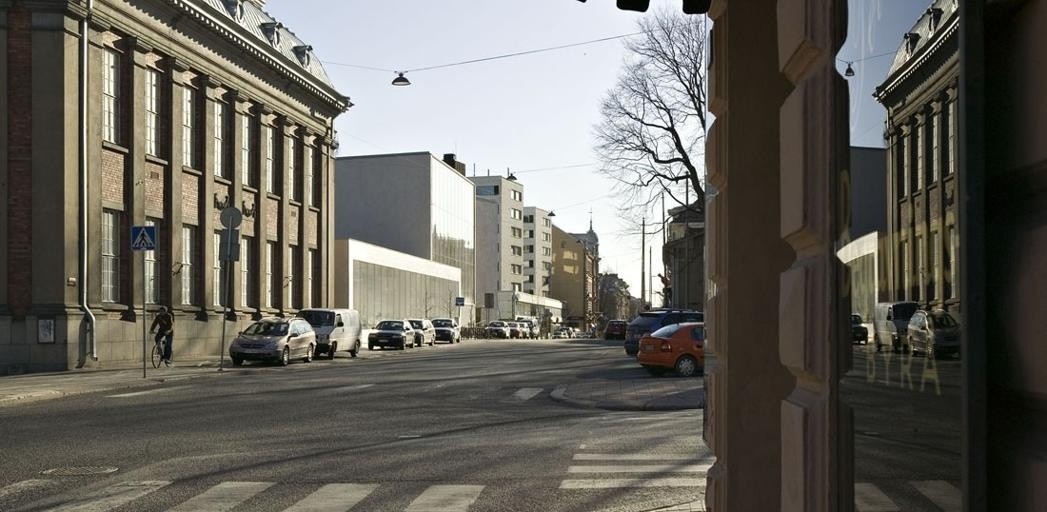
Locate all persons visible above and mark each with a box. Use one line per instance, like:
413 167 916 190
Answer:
252 324 271 335
267 323 282 335
148 305 174 363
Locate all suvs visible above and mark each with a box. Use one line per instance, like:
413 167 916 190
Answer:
623 307 702 357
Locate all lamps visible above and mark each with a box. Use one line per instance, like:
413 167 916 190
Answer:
392 72 411 86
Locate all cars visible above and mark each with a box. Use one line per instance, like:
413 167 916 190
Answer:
367 320 415 351
906 309 959 360
229 316 316 366
405 318 435 347
635 317 703 377
850 314 867 345
484 318 598 338
429 318 461 344
602 320 629 340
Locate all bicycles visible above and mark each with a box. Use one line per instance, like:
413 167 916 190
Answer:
148 330 174 369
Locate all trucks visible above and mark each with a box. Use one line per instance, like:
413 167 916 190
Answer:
294 308 361 360
872 301 921 352
